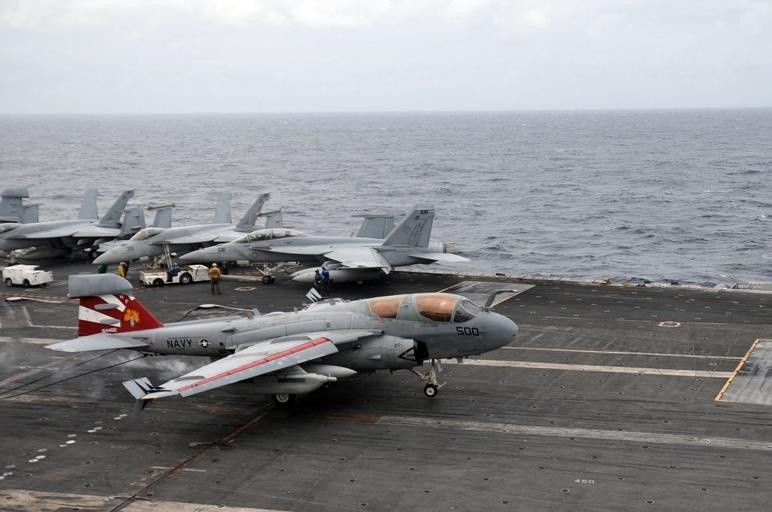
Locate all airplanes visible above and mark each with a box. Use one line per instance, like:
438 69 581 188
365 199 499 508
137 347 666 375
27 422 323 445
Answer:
41 272 521 419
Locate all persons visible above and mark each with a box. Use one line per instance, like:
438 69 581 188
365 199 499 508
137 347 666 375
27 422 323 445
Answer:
209 263 222 295
315 266 332 296
118 262 130 277
98 265 107 273
168 263 181 282
